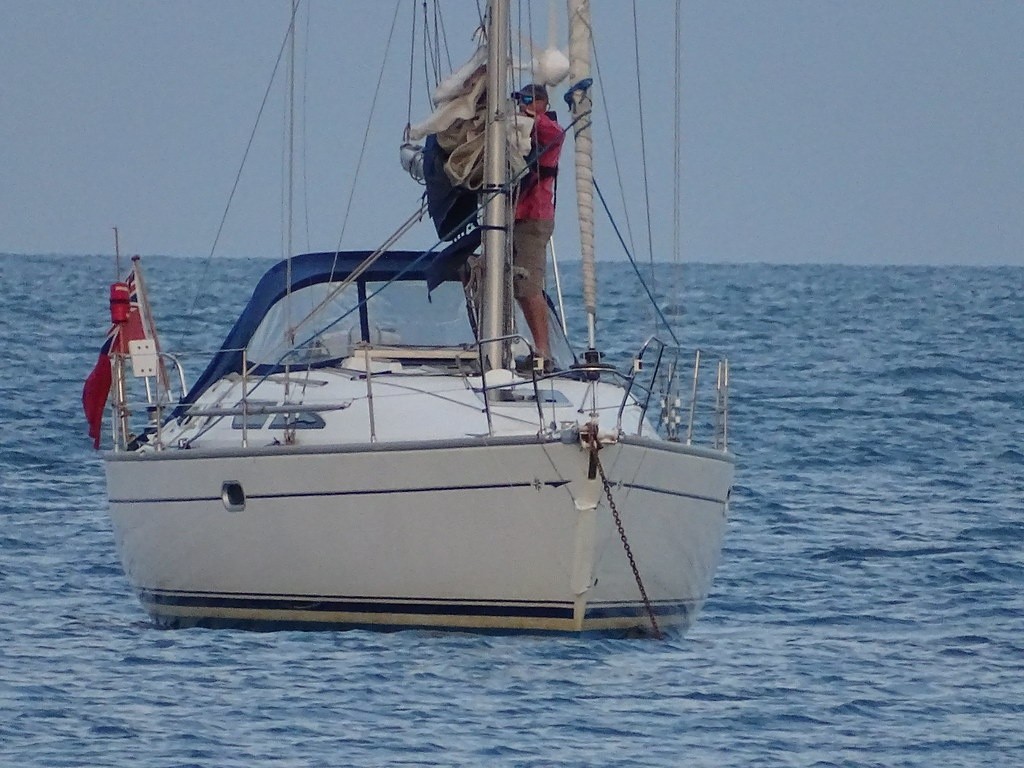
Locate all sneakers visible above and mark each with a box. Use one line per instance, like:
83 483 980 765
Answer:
515 356 554 373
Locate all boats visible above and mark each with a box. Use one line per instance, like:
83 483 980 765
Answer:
100 1 737 634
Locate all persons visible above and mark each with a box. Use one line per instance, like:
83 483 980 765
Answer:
509 83 566 376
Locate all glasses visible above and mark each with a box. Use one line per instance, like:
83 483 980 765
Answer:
514 96 544 105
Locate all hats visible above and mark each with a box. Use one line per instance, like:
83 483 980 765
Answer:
511 84 547 99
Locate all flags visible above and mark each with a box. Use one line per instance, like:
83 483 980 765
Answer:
83 266 148 452
